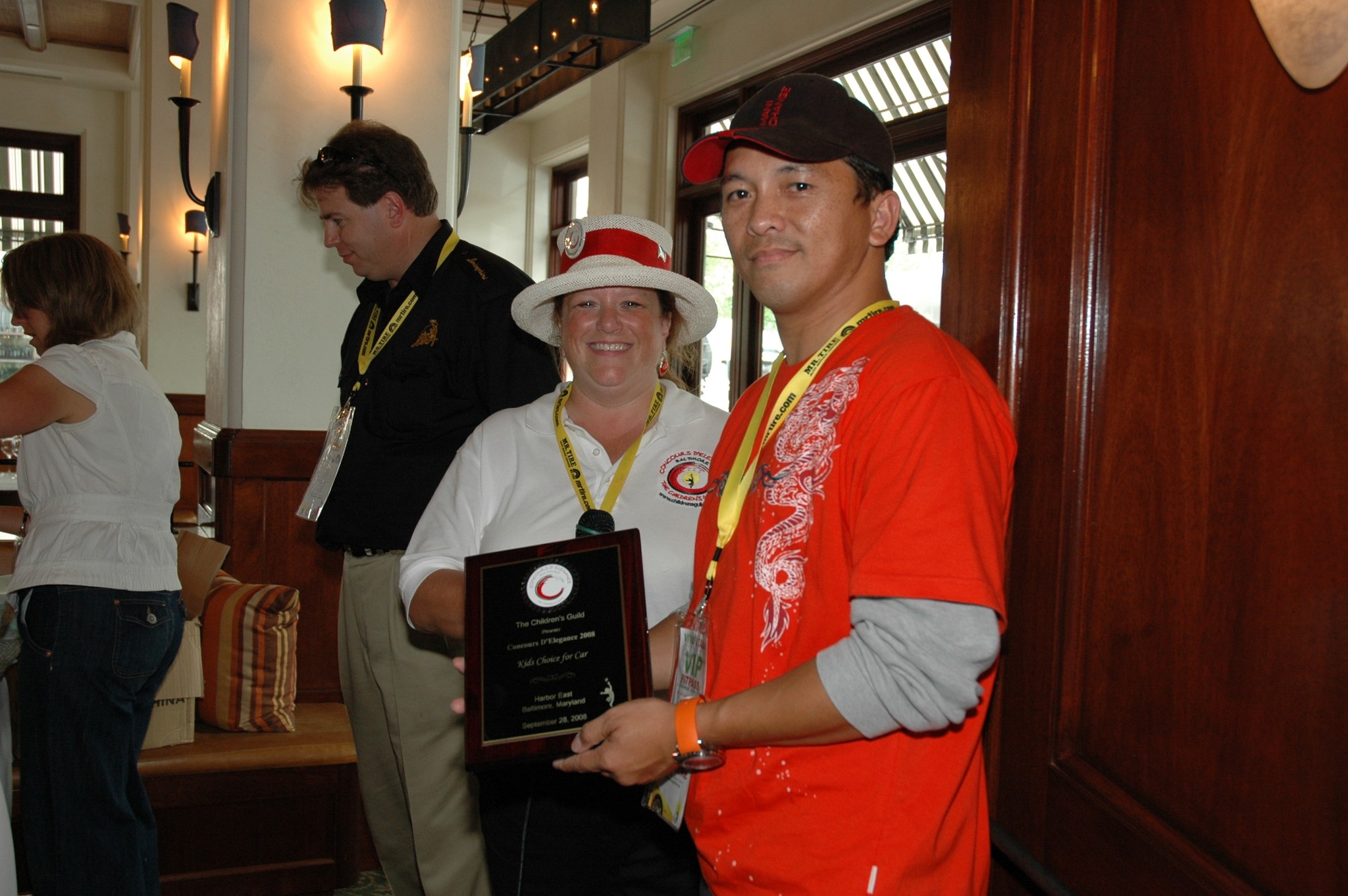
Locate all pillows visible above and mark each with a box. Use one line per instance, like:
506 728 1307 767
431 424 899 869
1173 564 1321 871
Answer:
203 568 303 731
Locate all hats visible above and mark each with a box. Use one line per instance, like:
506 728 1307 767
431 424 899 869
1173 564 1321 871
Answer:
681 73 895 185
511 214 718 350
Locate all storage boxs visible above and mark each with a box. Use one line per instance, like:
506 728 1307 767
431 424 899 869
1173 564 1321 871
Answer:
141 531 231 750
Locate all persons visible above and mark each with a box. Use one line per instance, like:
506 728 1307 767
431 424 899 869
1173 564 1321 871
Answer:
452 76 1018 896
290 119 567 896
1 234 187 896
401 217 732 631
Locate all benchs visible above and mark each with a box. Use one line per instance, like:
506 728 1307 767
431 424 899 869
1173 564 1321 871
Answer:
14 701 372 896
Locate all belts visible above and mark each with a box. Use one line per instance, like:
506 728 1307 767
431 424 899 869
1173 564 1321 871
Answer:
338 545 392 558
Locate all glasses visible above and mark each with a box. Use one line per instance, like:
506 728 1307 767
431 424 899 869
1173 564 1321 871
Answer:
316 145 395 178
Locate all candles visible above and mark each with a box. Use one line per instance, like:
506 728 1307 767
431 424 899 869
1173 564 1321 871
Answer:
499 89 505 97
180 60 191 97
194 232 199 250
570 43 578 51
462 82 472 127
531 68 539 76
552 54 558 61
484 99 491 106
514 79 522 87
123 238 128 251
352 45 361 85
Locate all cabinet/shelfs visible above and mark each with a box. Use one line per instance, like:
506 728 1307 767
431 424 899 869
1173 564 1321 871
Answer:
0 332 38 380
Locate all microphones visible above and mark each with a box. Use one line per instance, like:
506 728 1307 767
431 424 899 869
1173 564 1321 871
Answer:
576 508 615 539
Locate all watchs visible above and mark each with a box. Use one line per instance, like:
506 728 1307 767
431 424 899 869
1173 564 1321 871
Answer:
672 695 725 772
